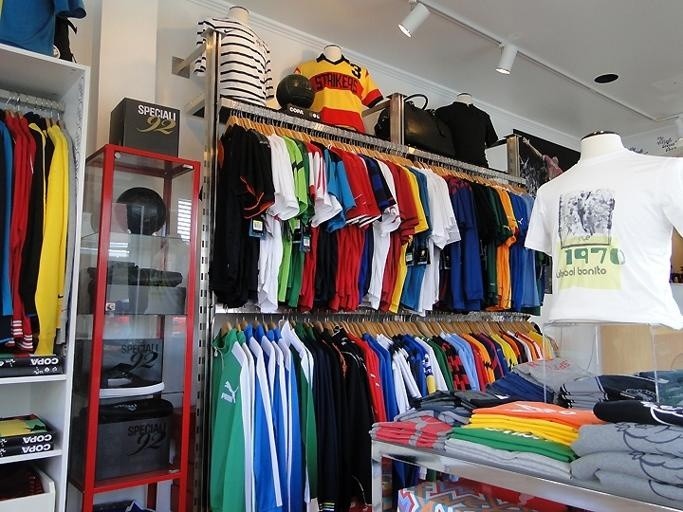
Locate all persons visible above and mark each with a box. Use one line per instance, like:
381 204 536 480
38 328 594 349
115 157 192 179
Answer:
192 6 274 107
435 93 498 169
525 131 683 331
294 45 383 133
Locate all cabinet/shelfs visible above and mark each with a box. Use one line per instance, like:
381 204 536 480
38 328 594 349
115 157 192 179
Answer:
370 439 679 510
67 145 202 512
1 41 92 511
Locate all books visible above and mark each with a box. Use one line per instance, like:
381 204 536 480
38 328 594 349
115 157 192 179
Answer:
0 353 64 377
0 413 56 458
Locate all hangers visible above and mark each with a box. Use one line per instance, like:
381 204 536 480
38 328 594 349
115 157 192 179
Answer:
220 101 534 195
216 306 545 340
2 87 68 130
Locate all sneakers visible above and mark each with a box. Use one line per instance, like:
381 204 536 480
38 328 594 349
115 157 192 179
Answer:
99 372 165 398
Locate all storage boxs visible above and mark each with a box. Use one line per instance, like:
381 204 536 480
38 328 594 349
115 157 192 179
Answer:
110 98 181 159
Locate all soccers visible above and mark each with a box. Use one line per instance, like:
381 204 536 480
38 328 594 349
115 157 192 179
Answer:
276 75 314 108
116 187 166 235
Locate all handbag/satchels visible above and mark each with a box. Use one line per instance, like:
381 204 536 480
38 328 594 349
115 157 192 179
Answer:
71 399 173 481
375 93 455 160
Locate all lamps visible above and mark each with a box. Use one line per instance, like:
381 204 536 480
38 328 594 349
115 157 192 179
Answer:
398 1 520 75
